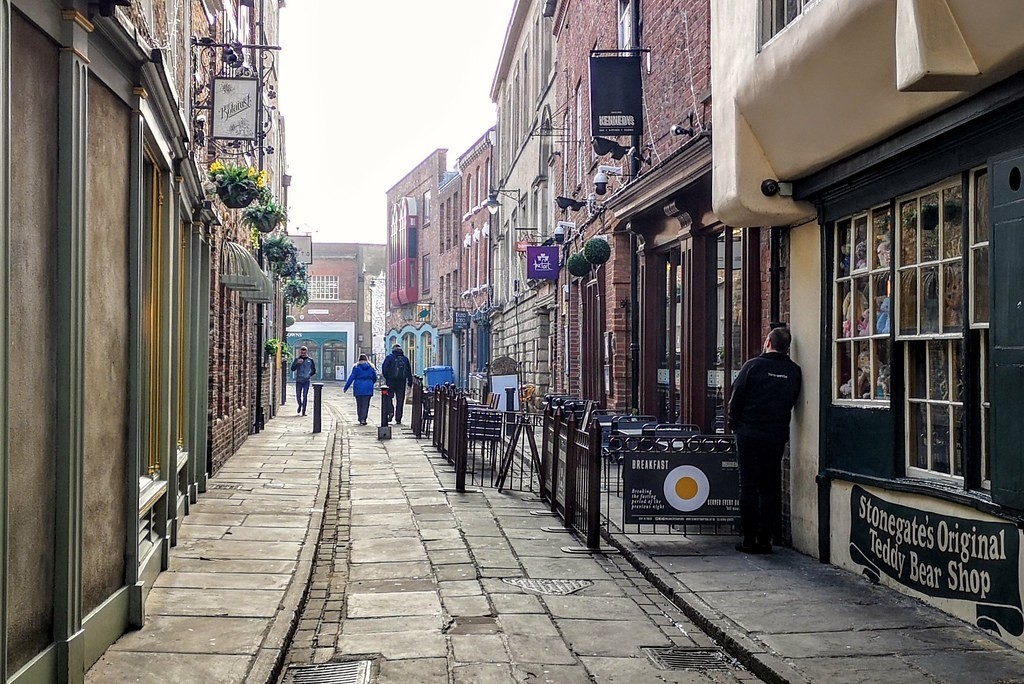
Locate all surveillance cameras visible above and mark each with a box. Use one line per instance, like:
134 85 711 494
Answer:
761 179 779 197
554 227 564 243
593 173 608 195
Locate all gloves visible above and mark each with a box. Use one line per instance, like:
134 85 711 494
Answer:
408 380 412 387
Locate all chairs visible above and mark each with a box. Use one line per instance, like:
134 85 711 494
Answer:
419 387 500 473
544 392 701 498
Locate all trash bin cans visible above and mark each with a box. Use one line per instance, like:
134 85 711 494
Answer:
423 365 453 390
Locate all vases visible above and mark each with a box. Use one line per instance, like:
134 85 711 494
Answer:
286 268 297 277
254 219 277 233
292 292 301 298
215 181 253 208
274 254 286 262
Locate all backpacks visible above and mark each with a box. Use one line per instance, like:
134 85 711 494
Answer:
393 353 406 381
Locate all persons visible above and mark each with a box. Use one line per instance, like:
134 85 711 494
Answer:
726 326 803 557
382 344 414 424
290 346 317 417
343 354 380 427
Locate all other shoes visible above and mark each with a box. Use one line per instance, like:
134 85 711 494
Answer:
397 420 401 424
303 414 307 416
388 413 393 422
735 540 772 554
360 421 367 426
297 404 301 413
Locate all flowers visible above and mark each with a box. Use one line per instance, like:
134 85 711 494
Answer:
206 160 271 206
260 228 312 308
238 195 292 231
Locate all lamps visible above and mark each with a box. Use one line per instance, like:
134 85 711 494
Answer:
594 165 624 197
554 220 576 245
591 136 631 160
555 196 586 212
542 237 554 247
670 123 693 137
627 146 651 166
485 95 573 214
